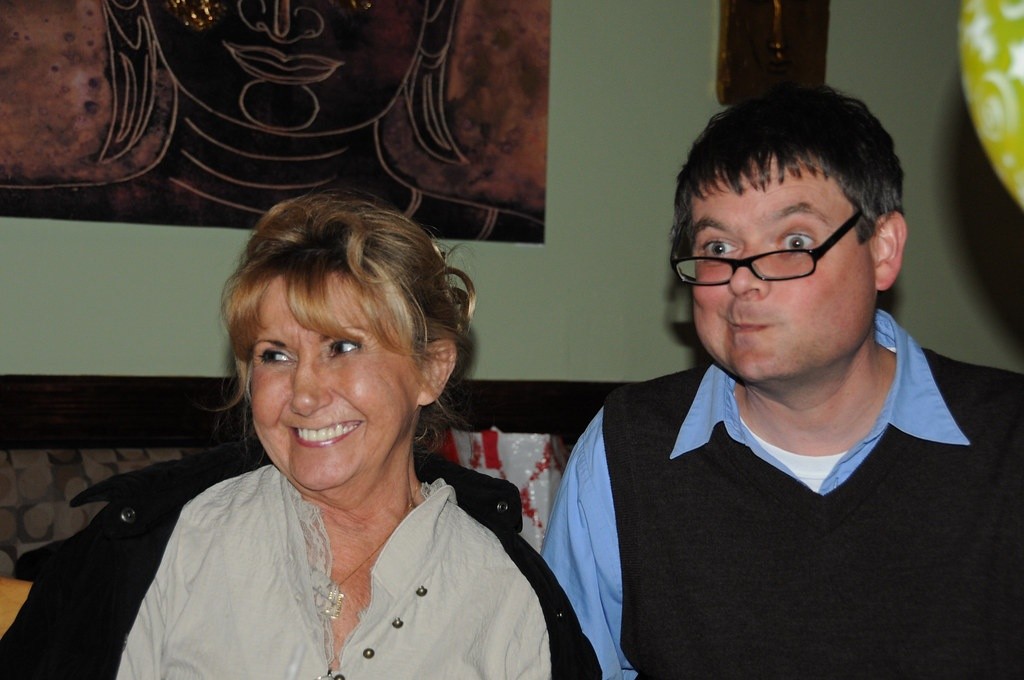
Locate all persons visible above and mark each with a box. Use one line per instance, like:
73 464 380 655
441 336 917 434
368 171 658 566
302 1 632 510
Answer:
0 193 603 680
540 93 1024 680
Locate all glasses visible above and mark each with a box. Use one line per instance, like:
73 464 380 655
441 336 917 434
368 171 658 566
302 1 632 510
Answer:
669 208 863 287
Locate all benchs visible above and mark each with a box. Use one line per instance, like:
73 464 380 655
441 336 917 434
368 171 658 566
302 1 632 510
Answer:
0 375 635 637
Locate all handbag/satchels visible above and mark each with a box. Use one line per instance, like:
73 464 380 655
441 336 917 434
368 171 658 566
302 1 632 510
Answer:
427 426 568 557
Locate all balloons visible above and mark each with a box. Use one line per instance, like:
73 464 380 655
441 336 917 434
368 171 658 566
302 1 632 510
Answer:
959 0 1024 205
